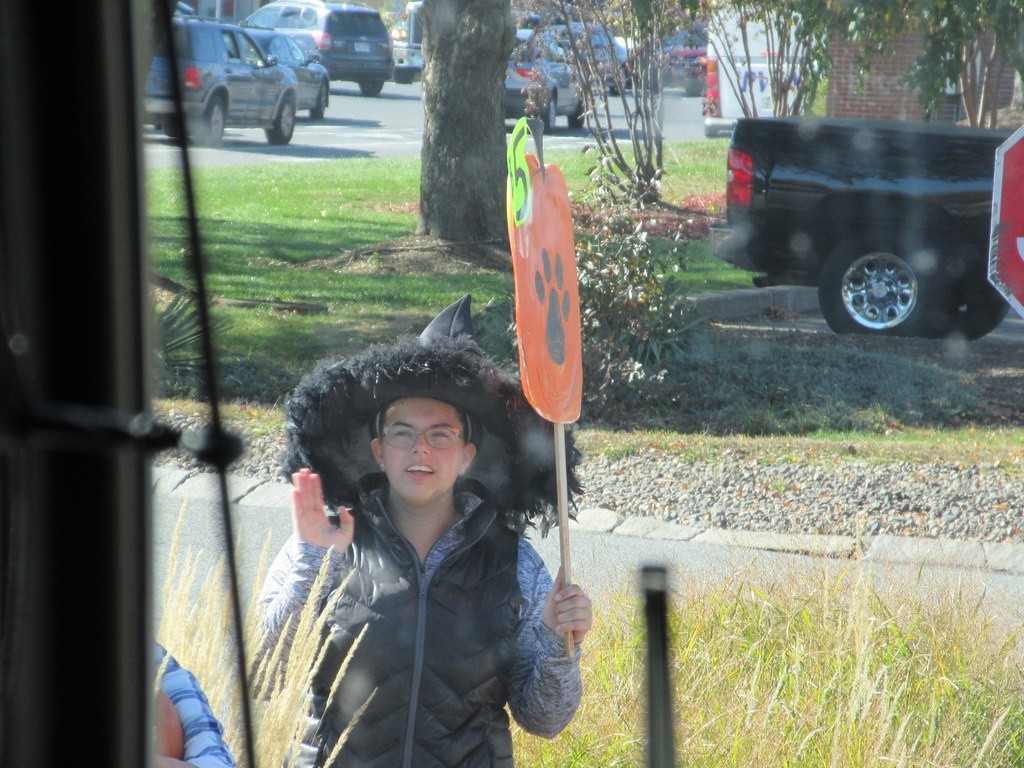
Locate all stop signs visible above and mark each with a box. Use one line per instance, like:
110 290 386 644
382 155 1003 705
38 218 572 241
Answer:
989 124 1024 317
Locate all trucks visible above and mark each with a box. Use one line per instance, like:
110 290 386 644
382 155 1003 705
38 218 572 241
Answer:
382 0 425 85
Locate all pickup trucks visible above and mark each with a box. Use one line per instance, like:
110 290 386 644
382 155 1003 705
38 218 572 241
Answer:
714 116 1024 337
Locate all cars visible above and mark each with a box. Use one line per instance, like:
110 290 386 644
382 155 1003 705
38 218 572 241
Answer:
224 29 329 118
498 10 629 131
659 15 709 98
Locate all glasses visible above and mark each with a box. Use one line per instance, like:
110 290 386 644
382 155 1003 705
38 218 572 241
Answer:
375 421 468 450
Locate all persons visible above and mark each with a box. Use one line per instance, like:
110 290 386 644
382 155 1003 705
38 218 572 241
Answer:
248 294 592 768
150 639 237 768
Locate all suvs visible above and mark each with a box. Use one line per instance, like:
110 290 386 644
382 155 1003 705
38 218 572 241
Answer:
143 15 299 146
239 1 394 96
